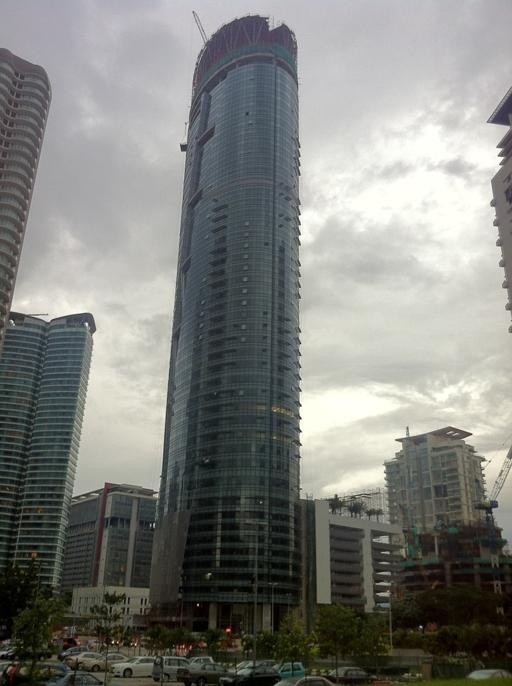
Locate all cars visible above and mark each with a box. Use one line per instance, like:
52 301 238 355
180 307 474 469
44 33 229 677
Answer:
464 669 512 681
152 656 378 686
399 673 422 682
0 636 156 686
